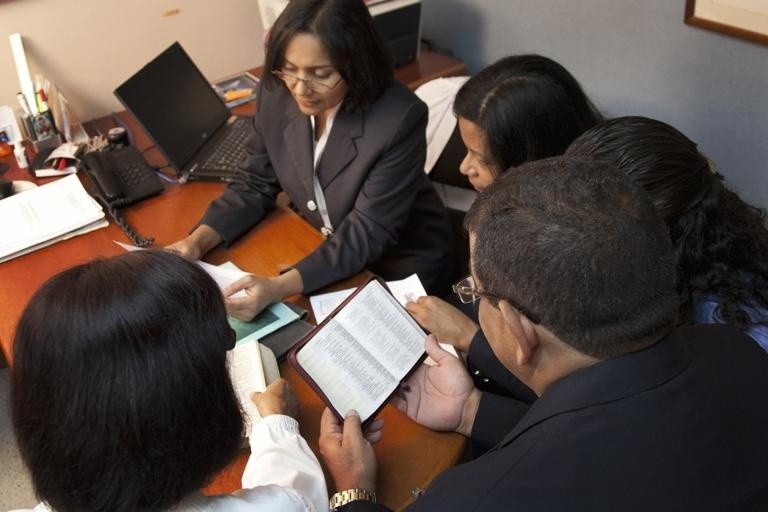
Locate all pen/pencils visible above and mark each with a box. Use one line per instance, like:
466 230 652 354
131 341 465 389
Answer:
17 92 33 117
58 158 67 172
38 89 48 110
51 158 58 168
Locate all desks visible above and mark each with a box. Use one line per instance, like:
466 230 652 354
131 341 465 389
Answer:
0 48 468 511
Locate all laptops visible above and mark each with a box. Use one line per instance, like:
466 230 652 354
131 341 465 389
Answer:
112 40 254 183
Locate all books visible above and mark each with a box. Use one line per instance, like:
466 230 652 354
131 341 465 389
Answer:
219 335 282 444
27 140 82 180
290 278 438 427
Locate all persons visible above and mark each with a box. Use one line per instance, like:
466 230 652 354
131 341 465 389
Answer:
1 248 330 512
560 115 767 350
403 53 606 408
319 156 767 511
163 1 461 324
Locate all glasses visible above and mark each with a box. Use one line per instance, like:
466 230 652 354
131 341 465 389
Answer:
454 276 542 325
270 65 343 93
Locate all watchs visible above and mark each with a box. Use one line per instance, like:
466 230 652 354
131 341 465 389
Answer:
327 487 378 508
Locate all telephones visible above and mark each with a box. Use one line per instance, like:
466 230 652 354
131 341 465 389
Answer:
80 144 164 248
